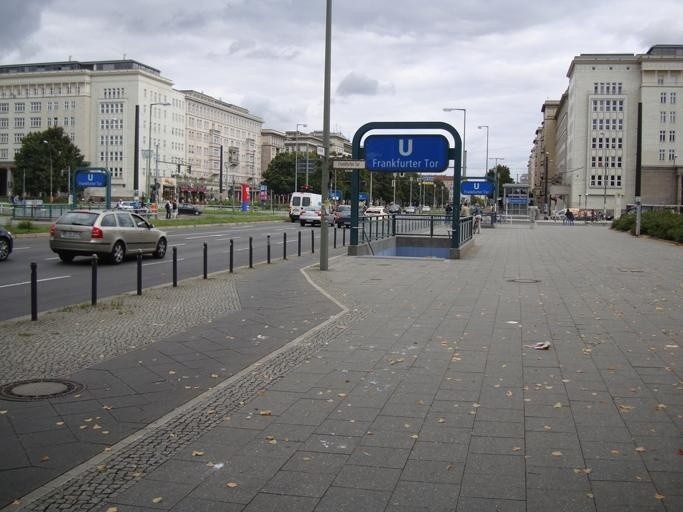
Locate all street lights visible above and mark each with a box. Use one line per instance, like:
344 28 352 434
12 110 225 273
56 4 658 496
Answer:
443 107 467 179
44 141 53 203
477 125 489 178
294 122 308 192
145 102 172 200
489 157 505 211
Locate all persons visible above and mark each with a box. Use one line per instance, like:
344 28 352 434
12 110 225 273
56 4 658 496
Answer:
443 202 481 235
116 199 123 210
164 200 171 219
564 208 573 221
362 204 367 215
172 200 177 218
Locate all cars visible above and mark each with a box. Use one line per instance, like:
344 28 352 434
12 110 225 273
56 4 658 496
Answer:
178 203 202 216
290 191 433 228
0 227 15 262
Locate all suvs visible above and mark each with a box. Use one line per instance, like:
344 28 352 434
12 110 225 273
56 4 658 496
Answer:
48 208 168 265
